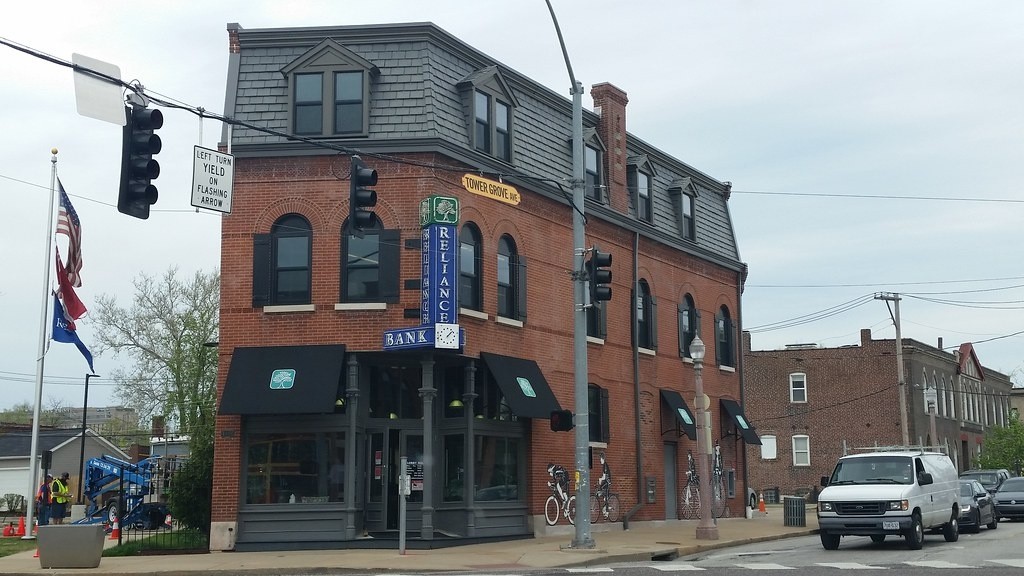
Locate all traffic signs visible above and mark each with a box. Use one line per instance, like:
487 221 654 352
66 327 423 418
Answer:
191 144 236 216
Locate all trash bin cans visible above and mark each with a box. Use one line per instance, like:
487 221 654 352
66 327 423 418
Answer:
781 496 807 526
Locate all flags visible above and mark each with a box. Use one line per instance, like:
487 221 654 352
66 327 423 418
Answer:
55 245 92 331
51 290 96 374
53 169 91 286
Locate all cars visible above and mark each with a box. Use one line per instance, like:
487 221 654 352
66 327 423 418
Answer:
748 487 757 509
954 479 997 534
994 477 1024 522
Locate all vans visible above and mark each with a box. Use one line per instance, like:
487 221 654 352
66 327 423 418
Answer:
815 451 963 551
957 467 1010 502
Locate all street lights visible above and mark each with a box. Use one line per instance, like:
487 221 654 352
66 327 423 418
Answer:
75 374 101 504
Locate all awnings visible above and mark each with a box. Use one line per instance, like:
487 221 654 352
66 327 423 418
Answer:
478 350 569 420
657 388 697 442
212 343 348 418
719 399 764 447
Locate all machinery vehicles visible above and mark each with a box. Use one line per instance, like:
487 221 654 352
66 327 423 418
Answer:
69 454 173 530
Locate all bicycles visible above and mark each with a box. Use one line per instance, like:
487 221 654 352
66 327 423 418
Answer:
545 482 575 525
590 485 620 524
680 473 701 519
711 472 727 518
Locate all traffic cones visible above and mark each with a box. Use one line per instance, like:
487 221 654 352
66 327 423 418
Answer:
14 516 25 536
108 516 123 539
34 518 39 533
33 548 39 557
758 493 766 513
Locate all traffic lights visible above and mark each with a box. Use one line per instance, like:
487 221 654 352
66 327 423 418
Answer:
591 244 612 305
348 155 379 240
116 104 164 219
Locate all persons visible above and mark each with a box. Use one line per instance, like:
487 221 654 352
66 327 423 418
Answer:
34 473 54 526
52 472 74 525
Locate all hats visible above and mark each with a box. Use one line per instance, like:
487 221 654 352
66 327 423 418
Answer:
46 473 53 479
61 471 70 480
